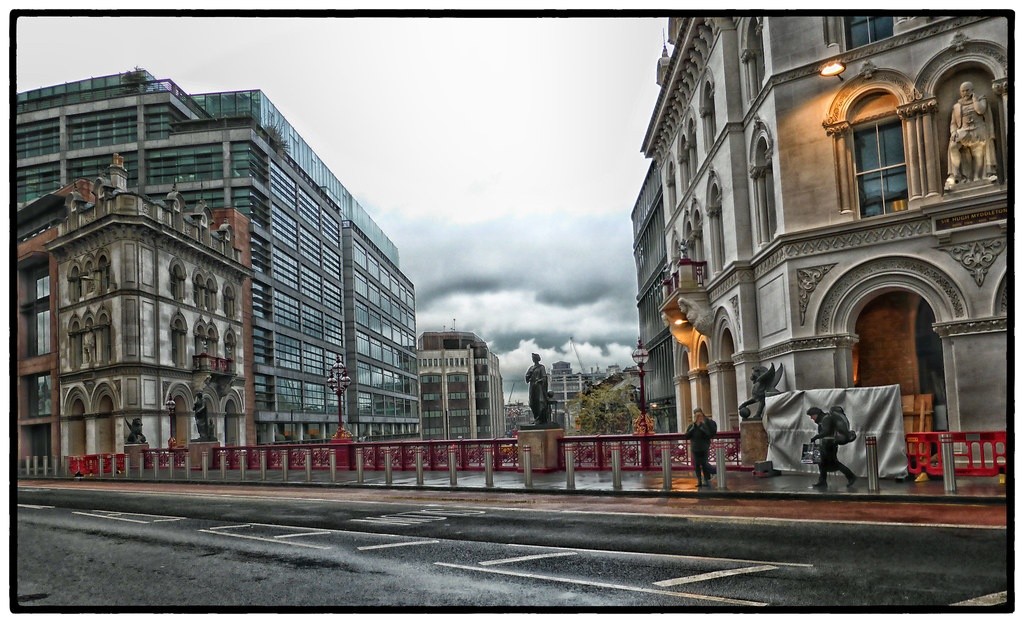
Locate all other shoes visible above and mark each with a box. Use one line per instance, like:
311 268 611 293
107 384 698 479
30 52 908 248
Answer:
813 481 827 487
702 481 708 485
695 481 702 487
847 475 857 487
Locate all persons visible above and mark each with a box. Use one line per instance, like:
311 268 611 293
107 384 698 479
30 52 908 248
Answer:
83 324 95 363
944 82 998 190
685 408 716 487
525 353 552 423
168 434 177 448
807 407 857 487
192 392 209 439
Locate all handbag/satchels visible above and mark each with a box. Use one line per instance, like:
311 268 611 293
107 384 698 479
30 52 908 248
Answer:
801 441 824 465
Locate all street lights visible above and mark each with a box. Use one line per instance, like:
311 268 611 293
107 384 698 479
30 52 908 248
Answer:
328 354 353 443
166 392 176 450
631 338 653 434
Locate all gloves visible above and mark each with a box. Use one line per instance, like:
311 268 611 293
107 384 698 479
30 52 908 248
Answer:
811 438 816 443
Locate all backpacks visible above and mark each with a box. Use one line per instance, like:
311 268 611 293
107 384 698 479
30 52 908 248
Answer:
822 406 856 444
708 420 716 437
